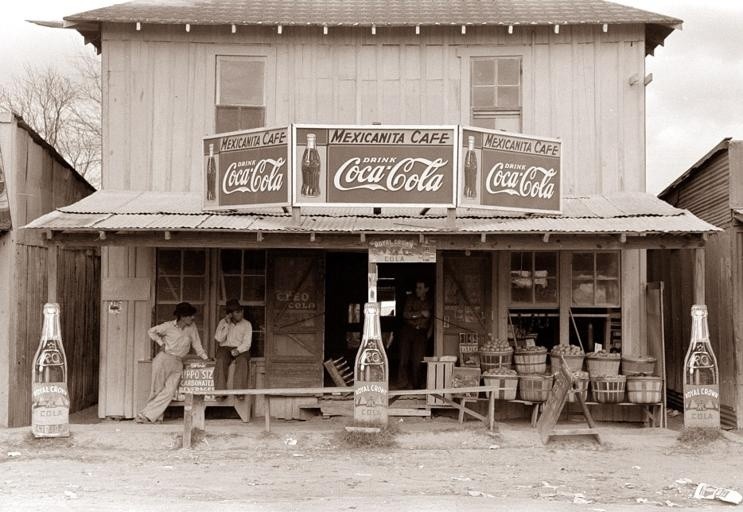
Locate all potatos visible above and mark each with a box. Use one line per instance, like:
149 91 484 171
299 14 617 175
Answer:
484 365 518 376
479 333 514 352
515 345 545 352
550 343 584 356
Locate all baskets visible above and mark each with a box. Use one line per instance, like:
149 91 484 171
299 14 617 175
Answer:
479 350 663 404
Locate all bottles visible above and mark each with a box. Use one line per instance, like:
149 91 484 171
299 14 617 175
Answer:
465 136 477 200
30 301 69 440
350 302 390 434
298 133 321 198
681 304 719 433
204 144 218 205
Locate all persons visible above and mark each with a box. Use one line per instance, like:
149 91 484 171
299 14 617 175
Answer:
214 299 253 401
389 278 431 390
135 302 213 424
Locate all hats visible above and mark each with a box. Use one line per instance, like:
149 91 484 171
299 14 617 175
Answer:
173 302 197 316
225 298 243 314
